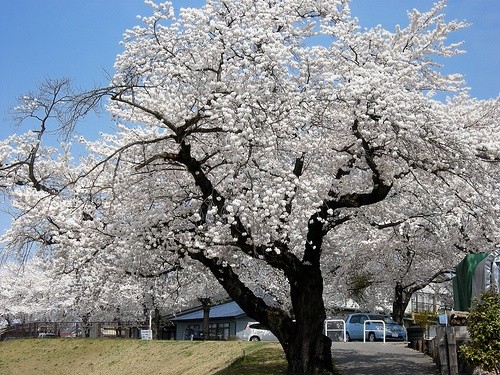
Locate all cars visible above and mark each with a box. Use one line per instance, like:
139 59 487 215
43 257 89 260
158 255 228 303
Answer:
243 322 280 341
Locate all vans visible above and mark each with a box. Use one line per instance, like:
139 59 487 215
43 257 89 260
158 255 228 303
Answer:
342 313 405 341
